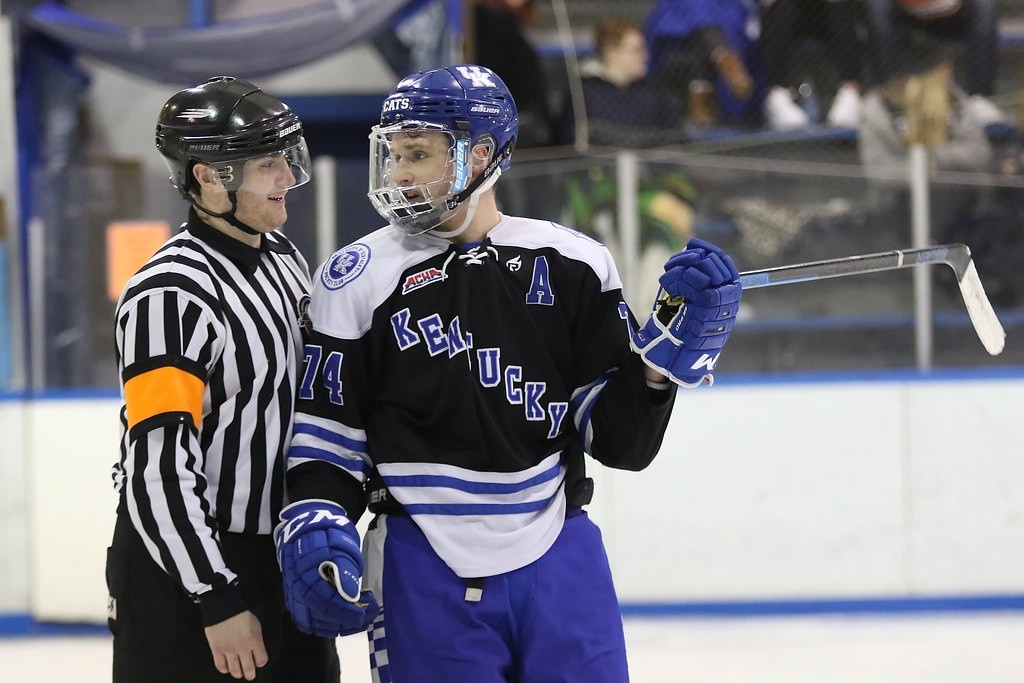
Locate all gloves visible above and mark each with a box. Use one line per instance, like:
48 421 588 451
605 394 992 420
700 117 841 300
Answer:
627 236 739 390
273 500 381 641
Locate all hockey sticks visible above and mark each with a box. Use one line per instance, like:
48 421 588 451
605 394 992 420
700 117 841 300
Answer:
738 242 1008 357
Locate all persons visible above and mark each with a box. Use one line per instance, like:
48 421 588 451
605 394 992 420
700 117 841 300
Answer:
471 0 1024 311
105 75 342 682
274 61 740 682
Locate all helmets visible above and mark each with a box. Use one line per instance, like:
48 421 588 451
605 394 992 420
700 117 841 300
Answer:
154 74 303 189
368 64 518 237
